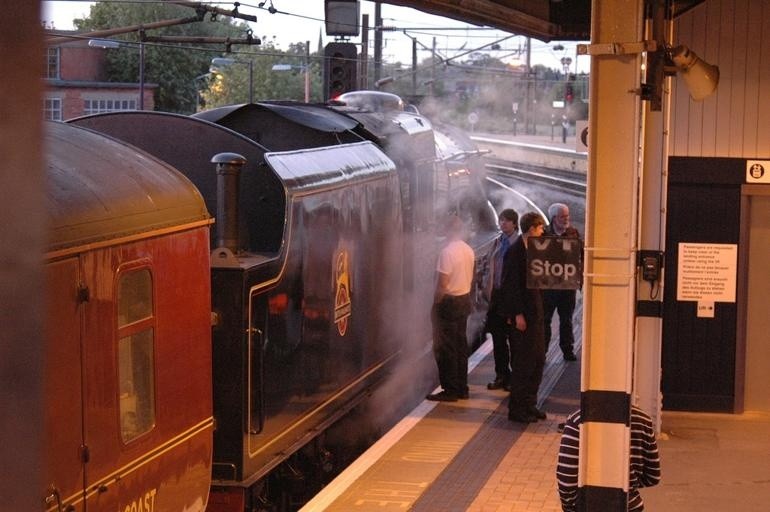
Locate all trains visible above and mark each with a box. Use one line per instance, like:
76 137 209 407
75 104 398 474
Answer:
37 91 505 511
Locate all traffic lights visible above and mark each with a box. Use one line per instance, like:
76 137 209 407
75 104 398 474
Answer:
321 40 357 105
325 1 360 36
566 85 574 102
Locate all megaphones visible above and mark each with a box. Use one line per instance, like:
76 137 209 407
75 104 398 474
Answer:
672 44 720 102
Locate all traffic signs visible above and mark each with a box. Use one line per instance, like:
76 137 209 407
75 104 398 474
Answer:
468 112 480 125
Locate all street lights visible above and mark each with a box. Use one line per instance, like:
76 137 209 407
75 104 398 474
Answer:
88 38 145 111
211 57 254 107
512 100 518 135
271 63 309 103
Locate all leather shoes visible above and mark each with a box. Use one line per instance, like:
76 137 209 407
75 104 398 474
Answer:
426 352 577 422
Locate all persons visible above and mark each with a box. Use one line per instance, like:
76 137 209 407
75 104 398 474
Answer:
486 209 521 392
541 204 583 361
504 212 545 424
426 215 476 402
557 407 661 511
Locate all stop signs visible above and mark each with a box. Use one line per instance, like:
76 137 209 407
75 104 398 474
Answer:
524 234 583 291
